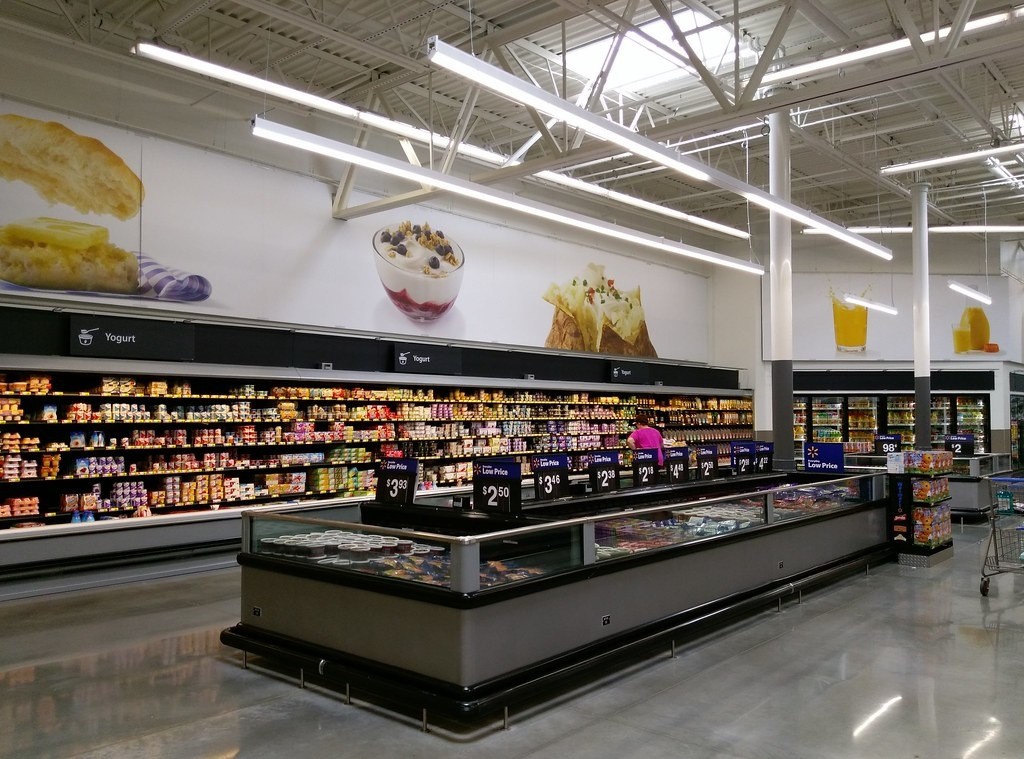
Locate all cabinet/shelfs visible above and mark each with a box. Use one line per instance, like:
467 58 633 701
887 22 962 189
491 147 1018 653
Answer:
887 471 955 568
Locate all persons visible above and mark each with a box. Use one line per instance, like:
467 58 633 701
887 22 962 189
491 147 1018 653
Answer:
627 415 664 467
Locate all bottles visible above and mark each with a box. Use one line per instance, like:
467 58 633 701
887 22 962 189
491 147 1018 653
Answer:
636 399 754 441
960 285 990 350
997 485 1013 516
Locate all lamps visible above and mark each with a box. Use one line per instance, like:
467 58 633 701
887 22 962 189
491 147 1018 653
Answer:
946 190 992 305
129 0 752 244
843 258 898 316
799 97 1024 236
250 0 765 276
426 0 893 263
869 91 1024 177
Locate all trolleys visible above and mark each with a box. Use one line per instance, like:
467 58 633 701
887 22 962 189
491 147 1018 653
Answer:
980 468 1024 596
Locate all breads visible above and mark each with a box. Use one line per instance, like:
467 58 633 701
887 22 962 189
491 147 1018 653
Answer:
0 112 145 221
0 226 140 295
538 262 658 357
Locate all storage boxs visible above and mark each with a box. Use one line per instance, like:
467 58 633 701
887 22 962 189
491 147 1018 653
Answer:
596 518 695 544
887 449 953 550
0 375 618 513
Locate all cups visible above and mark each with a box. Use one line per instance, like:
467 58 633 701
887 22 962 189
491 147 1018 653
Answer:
952 323 971 355
372 224 466 323
829 283 871 353
